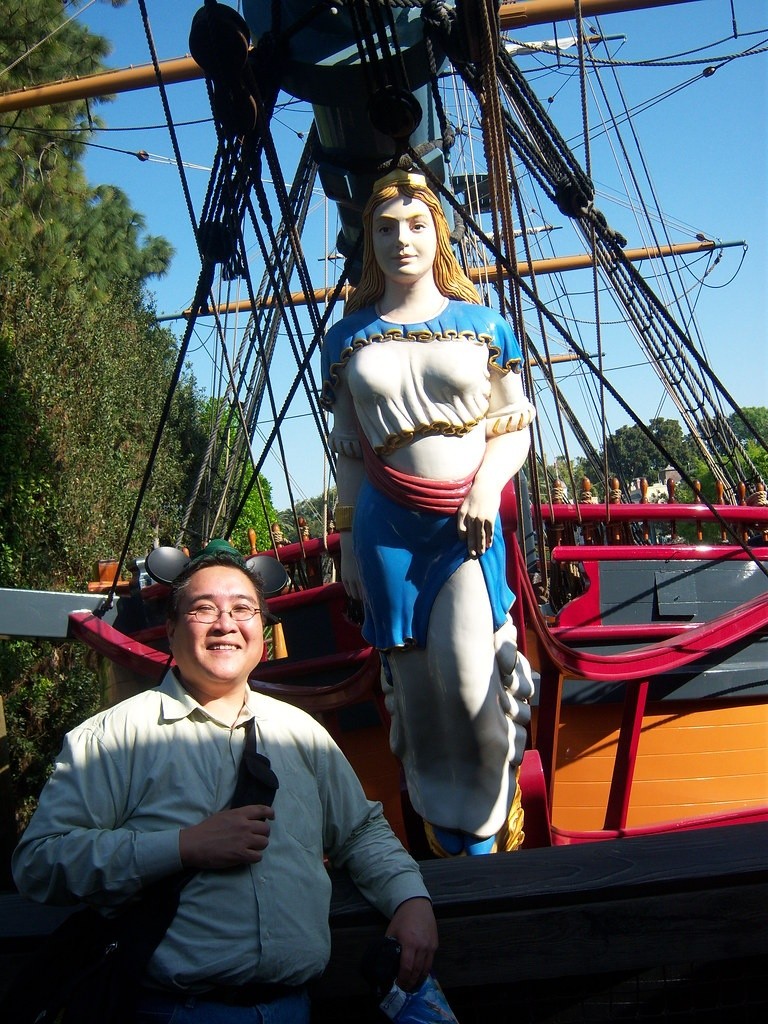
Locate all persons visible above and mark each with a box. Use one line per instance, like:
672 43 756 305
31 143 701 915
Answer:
318 168 538 857
11 557 439 1024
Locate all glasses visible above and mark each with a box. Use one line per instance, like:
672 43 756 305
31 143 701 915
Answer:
177 604 265 624
